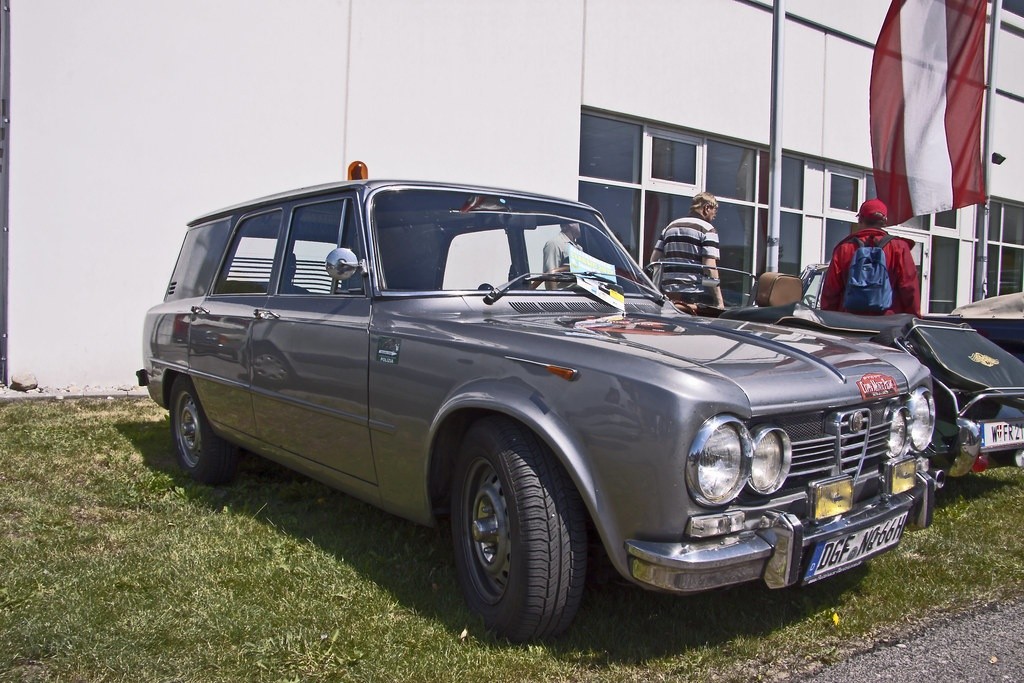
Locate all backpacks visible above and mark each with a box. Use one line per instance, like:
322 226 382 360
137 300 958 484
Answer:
842 235 898 314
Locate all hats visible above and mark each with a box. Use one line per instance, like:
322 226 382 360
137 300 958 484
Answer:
854 197 889 220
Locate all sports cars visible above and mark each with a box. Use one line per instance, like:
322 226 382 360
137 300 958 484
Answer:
632 261 1024 489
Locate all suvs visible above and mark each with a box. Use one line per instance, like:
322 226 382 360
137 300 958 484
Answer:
134 160 947 647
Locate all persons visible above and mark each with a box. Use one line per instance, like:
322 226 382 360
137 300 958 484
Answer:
542 222 581 291
649 191 725 311
820 201 922 318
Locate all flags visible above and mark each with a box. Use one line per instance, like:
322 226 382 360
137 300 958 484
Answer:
869 0 988 227
